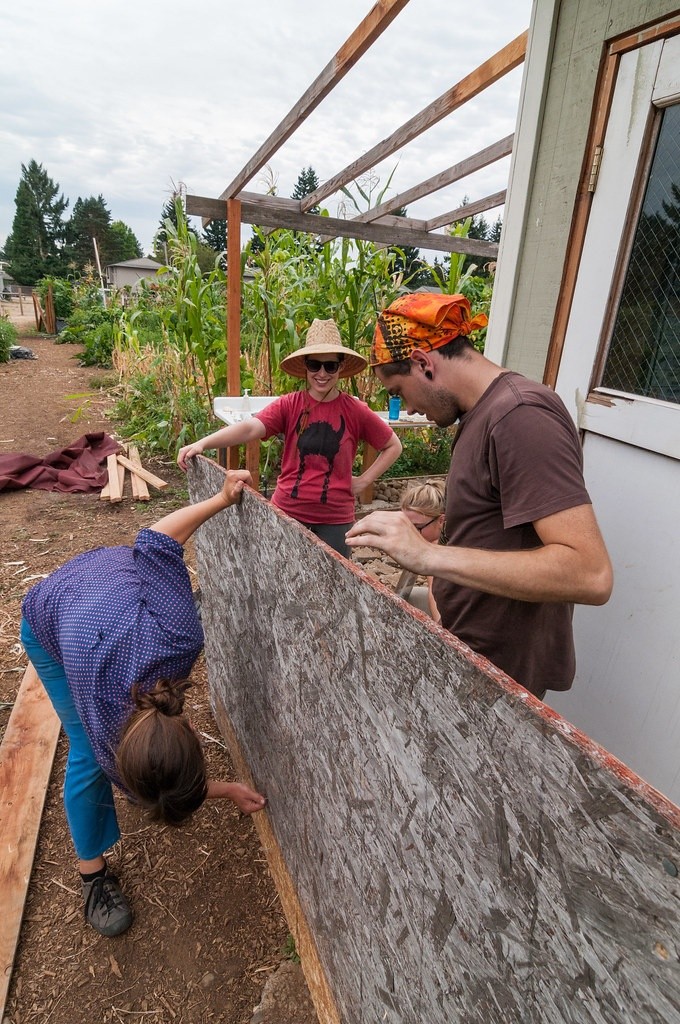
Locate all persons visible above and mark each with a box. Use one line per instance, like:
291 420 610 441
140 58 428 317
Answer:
400 478 445 623
177 319 403 560
20 469 265 938
343 292 614 703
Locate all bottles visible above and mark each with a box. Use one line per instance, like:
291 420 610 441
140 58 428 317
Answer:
388 396 400 420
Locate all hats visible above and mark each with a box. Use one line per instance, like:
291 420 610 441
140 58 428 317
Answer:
280 318 367 379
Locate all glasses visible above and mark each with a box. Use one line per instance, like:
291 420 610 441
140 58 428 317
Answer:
304 357 343 375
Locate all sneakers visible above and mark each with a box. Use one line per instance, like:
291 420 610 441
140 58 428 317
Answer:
77 857 132 933
